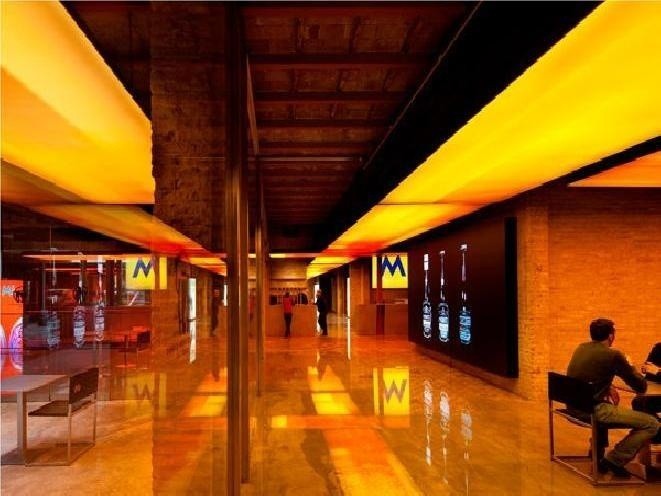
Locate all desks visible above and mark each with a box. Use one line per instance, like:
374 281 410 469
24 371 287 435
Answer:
0 375 70 465
612 376 661 423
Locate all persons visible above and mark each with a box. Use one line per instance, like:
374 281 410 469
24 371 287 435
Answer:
248 286 256 338
209 289 223 338
309 289 329 335
283 292 295 338
566 319 661 479
631 341 661 444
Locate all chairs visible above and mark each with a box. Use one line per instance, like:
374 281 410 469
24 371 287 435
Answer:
547 371 645 486
25 367 99 467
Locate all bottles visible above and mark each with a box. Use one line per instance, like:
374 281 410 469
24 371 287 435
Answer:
434 250 451 345
38 248 108 359
455 243 476 352
418 379 473 494
418 253 432 342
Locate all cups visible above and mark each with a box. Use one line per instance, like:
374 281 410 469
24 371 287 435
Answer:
644 360 656 365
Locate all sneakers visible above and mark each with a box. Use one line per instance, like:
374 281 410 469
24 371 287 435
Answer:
587 447 609 474
600 456 631 478
